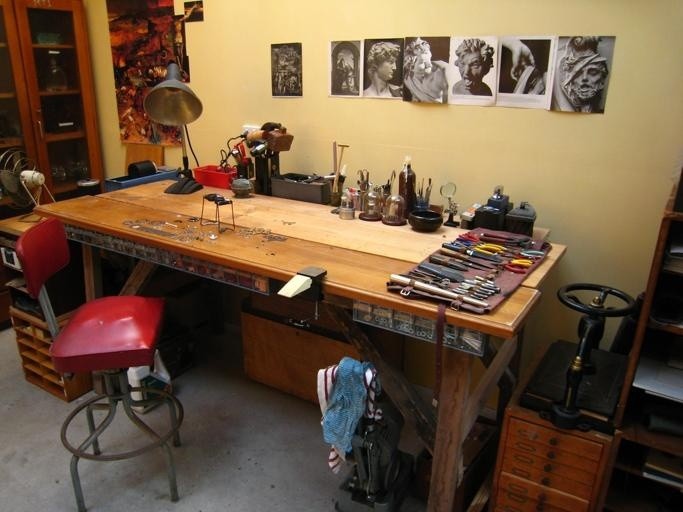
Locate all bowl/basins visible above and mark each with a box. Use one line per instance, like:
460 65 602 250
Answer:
408 211 443 233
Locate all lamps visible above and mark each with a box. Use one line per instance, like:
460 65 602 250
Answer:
143 63 203 194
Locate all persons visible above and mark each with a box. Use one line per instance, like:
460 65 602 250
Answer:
451 37 494 95
402 38 449 104
500 40 537 88
555 35 609 113
363 41 403 97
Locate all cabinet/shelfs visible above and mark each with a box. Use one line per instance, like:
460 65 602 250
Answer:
594 164 682 512
9 307 67 403
488 409 612 512
0 0 106 204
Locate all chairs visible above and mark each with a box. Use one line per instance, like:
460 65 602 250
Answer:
16 216 183 512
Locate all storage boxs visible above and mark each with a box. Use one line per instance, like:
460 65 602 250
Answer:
241 298 403 406
249 291 352 334
271 172 343 204
193 165 236 189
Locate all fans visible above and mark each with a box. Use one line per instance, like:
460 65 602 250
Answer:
0 148 46 206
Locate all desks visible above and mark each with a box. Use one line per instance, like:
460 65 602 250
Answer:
33 179 569 512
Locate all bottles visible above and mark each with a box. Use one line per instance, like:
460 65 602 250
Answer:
399 159 416 219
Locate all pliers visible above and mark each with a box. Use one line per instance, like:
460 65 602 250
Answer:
500 256 533 267
482 243 506 254
479 233 533 249
456 238 477 246
506 250 544 258
491 261 529 274
460 233 484 245
474 246 497 255
501 252 542 264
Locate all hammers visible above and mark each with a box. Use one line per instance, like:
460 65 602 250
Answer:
333 144 349 191
